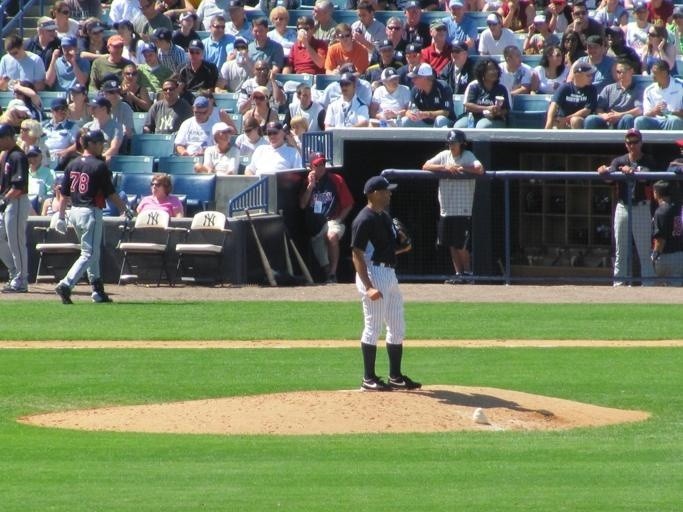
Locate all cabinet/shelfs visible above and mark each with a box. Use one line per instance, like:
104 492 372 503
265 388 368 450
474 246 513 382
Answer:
504 152 641 285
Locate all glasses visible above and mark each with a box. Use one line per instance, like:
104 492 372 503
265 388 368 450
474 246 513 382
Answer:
564 36 579 44
233 44 245 50
212 24 227 29
673 14 681 19
549 50 564 57
646 31 662 40
387 24 402 32
20 126 31 132
162 86 176 93
297 25 309 32
53 107 65 111
626 139 640 146
59 8 70 14
450 48 466 53
573 9 590 17
256 66 270 72
487 21 498 26
533 22 544 27
150 181 164 187
337 32 353 39
434 27 448 32
189 49 203 56
340 82 352 87
91 31 105 33
104 89 120 95
311 161 328 168
616 66 632 73
616 66 631 74
253 95 267 100
9 49 18 57
149 181 166 188
266 130 280 137
243 127 256 134
123 71 137 77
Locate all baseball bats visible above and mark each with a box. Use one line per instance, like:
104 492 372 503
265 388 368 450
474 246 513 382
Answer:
283 231 295 279
289 237 315 285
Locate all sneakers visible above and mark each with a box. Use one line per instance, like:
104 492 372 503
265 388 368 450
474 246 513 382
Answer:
452 275 477 284
386 374 423 391
0 278 28 294
360 377 394 392
91 287 112 303
442 274 464 283
324 271 339 285
55 284 75 305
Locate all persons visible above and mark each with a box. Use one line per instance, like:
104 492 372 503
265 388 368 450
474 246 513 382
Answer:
38 98 80 171
619 1 650 72
50 0 79 44
149 27 189 71
605 26 641 75
46 36 90 91
419 22 455 95
401 0 431 52
267 7 298 58
242 86 278 131
370 68 418 126
234 117 269 173
90 34 139 99
223 5 256 40
312 0 340 42
0 126 30 293
24 147 56 216
365 41 404 84
116 63 151 113
646 23 678 77
75 17 112 60
594 0 627 25
0 35 46 91
7 79 42 121
136 174 184 219
498 45 533 96
640 24 678 77
298 157 352 283
113 21 148 64
16 119 49 166
350 0 386 61
192 88 238 133
421 130 484 284
479 15 522 56
440 1 478 53
665 139 683 172
560 31 587 69
192 122 238 174
102 171 127 218
561 3 607 48
169 72 195 105
669 6 683 55
322 64 372 109
0 106 32 127
66 83 94 122
438 42 476 96
523 15 560 55
281 83 325 152
236 60 287 113
22 17 62 72
93 80 133 155
350 177 421 391
287 114 309 158
245 122 301 175
178 40 218 94
544 61 597 129
325 24 368 76
583 58 643 129
453 57 510 127
530 46 569 96
199 15 237 72
57 97 126 166
545 0 573 33
192 0 243 32
634 61 683 130
153 0 195 18
214 40 256 94
135 1 172 44
174 96 218 156
596 128 656 287
405 64 456 128
396 43 437 88
171 12 203 52
481 0 503 11
282 16 328 75
40 179 66 216
247 19 284 73
53 130 127 302
496 0 536 33
325 78 369 128
641 0 673 25
369 17 417 69
648 179 682 285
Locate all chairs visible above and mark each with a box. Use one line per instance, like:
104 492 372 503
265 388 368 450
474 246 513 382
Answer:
110 155 154 171
0 91 15 110
631 75 654 102
287 10 318 28
316 74 341 90
467 55 505 81
212 93 240 111
170 173 216 217
420 11 450 26
132 111 148 134
158 156 203 174
130 134 174 155
32 210 90 284
465 11 497 32
99 7 112 26
671 60 683 76
274 73 314 88
245 10 267 27
227 114 242 133
36 91 68 111
140 194 188 218
507 93 552 129
115 172 168 212
101 30 120 43
116 206 173 287
196 30 210 42
522 54 544 69
171 210 233 286
332 10 358 26
452 94 467 118
374 11 406 28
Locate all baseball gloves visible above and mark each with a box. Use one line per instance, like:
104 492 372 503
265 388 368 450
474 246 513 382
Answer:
123 208 138 222
52 218 71 235
243 206 279 287
0 193 10 214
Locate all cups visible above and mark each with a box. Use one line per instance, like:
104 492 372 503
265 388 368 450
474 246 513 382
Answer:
495 95 505 107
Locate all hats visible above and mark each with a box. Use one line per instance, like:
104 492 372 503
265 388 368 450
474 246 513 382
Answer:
113 18 134 30
233 36 248 51
106 34 127 46
674 139 683 148
605 23 626 37
8 97 31 113
49 97 69 113
572 60 599 77
252 86 271 101
632 0 647 15
534 13 547 25
485 12 504 25
86 95 112 108
363 174 399 195
449 1 467 7
178 9 197 24
338 61 363 78
192 96 209 107
150 27 172 42
336 71 356 86
177 10 199 23
585 35 604 48
265 120 284 133
672 4 683 17
59 35 78 48
211 120 237 138
188 38 204 53
68 82 86 97
37 14 58 32
446 40 470 51
141 41 158 54
100 79 123 94
429 18 451 31
380 67 402 82
405 43 421 53
446 128 468 143
625 127 641 142
243 116 259 134
406 62 435 78
81 130 109 145
307 149 329 165
229 0 244 11
84 21 105 34
403 0 420 11
23 143 41 158
377 38 393 50
0 123 17 136
